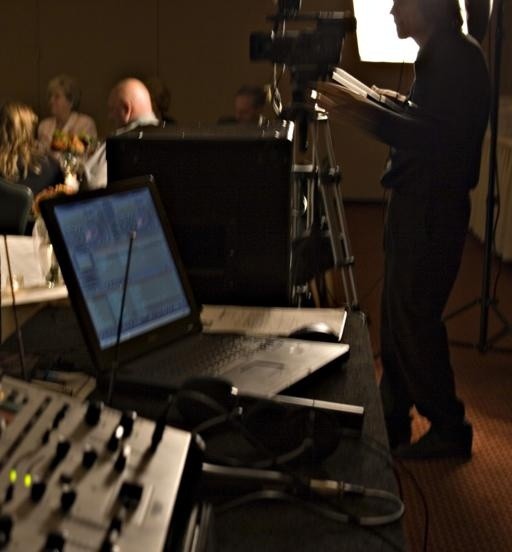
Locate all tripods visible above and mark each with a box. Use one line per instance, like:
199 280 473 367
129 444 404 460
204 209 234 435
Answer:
266 81 362 313
375 0 512 359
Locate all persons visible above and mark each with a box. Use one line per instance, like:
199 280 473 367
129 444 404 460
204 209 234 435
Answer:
0 102 61 235
216 85 265 126
314 0 493 461
65 77 159 193
38 75 97 152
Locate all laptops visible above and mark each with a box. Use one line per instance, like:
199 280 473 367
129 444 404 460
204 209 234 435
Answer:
38 174 353 402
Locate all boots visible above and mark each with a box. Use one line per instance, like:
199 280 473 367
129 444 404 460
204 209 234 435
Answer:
396 421 473 465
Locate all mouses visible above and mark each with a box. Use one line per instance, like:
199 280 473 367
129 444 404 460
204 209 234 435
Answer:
290 322 338 342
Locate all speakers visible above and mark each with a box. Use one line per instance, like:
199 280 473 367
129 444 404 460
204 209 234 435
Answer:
106 123 298 309
